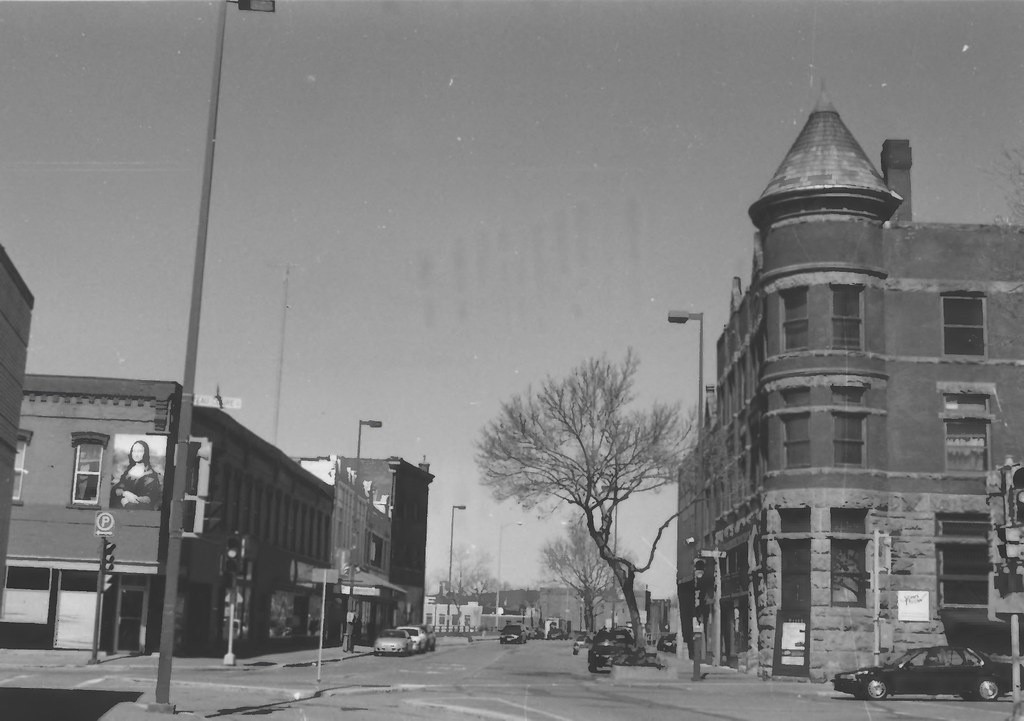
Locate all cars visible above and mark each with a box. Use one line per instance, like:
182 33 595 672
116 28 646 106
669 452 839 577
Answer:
396 626 427 654
593 628 634 654
526 626 545 639
547 628 568 640
574 636 588 648
835 644 1024 700
374 629 413 655
498 624 527 643
408 624 436 651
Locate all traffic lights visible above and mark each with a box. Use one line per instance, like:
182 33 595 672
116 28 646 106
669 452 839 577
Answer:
101 537 116 570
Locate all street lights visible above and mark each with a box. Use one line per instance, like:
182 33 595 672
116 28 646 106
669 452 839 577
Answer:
153 0 277 698
446 506 466 631
495 522 522 627
602 485 618 628
668 310 705 681
343 419 382 653
